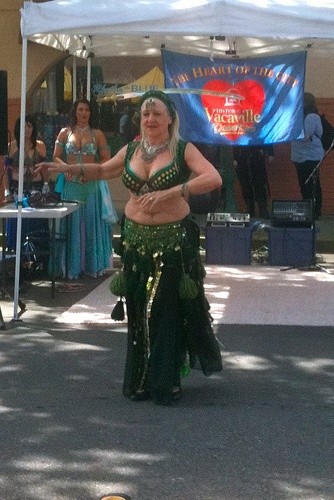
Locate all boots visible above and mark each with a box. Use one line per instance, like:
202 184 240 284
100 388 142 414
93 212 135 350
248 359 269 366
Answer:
256 197 270 219
245 198 254 217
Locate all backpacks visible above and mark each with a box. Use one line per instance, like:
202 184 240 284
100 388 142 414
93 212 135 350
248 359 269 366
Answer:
305 112 334 151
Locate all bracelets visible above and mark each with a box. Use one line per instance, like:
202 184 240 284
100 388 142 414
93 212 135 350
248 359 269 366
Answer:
4 189 10 196
79 164 86 176
181 181 191 203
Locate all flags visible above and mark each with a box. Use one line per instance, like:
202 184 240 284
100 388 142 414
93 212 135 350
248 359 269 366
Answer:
160 47 306 146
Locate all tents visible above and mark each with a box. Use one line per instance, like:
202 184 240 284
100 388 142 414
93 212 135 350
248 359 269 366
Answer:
13 0 334 320
96 66 164 130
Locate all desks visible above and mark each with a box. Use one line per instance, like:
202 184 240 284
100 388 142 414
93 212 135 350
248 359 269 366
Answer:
0 200 81 298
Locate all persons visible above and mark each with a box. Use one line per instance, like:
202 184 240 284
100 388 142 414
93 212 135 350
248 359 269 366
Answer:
232 144 274 218
33 89 223 404
2 98 113 280
291 92 324 217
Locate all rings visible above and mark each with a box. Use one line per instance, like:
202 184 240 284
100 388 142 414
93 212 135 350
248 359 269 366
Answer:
149 197 152 201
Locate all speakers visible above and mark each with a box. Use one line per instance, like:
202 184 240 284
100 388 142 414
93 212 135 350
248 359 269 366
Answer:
270 199 313 227
0 70 8 154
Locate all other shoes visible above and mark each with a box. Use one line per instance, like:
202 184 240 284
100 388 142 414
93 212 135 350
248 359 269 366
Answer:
173 385 181 400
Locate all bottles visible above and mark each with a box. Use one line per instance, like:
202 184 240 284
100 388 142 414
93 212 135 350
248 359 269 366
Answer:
42 182 50 195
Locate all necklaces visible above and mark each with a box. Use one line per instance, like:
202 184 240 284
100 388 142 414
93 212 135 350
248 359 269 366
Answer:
141 137 170 160
76 125 89 131
